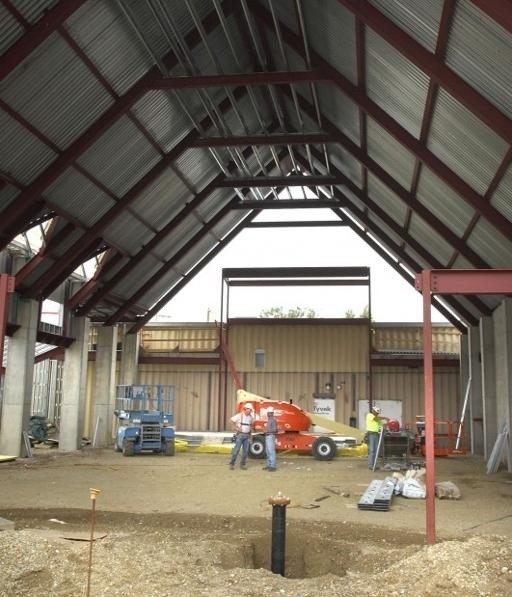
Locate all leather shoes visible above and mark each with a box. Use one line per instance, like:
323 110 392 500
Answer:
229 464 234 470
268 468 276 471
240 465 247 470
369 466 373 470
262 467 269 470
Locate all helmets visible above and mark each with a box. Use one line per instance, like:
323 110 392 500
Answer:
244 403 252 410
266 407 274 413
372 406 380 414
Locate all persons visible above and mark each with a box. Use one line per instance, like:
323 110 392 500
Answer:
227 403 254 470
365 407 390 469
263 407 278 472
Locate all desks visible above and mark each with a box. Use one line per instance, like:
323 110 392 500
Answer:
382 435 410 466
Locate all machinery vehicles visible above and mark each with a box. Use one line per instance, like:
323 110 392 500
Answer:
230 386 363 461
113 383 176 455
